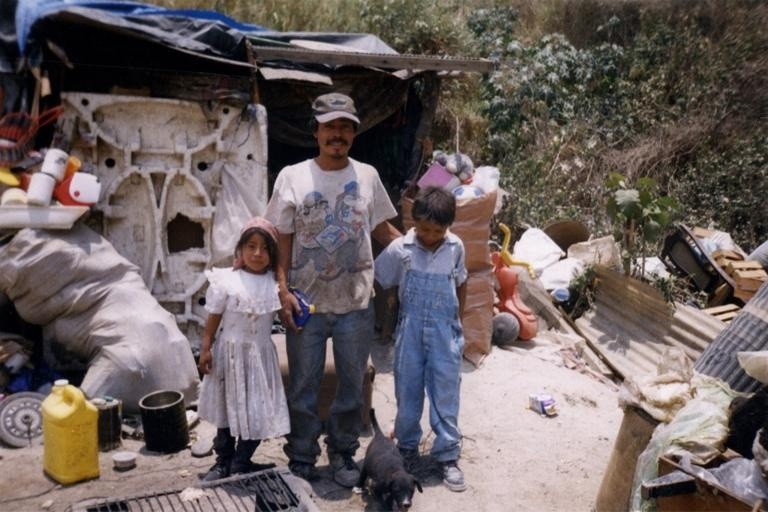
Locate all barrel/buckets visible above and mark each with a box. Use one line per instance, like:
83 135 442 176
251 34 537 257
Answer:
90 395 123 453
138 390 189 453
40 379 101 484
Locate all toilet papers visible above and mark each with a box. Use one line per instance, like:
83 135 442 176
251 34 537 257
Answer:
0 188 27 207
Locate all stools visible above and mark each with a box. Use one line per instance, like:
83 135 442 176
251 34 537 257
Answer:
267 333 375 427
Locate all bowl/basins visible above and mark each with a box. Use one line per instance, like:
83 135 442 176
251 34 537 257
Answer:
111 452 137 467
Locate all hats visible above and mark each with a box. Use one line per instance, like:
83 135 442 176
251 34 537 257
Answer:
240 216 281 250
310 93 361 129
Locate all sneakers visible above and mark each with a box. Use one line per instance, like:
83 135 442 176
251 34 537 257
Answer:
204 444 362 487
397 440 421 475
427 455 468 491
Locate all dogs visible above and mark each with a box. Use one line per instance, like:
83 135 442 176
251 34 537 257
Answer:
351 408 423 512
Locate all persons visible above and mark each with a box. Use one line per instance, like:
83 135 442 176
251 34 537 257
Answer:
198 218 291 484
373 183 467 492
262 93 404 488
